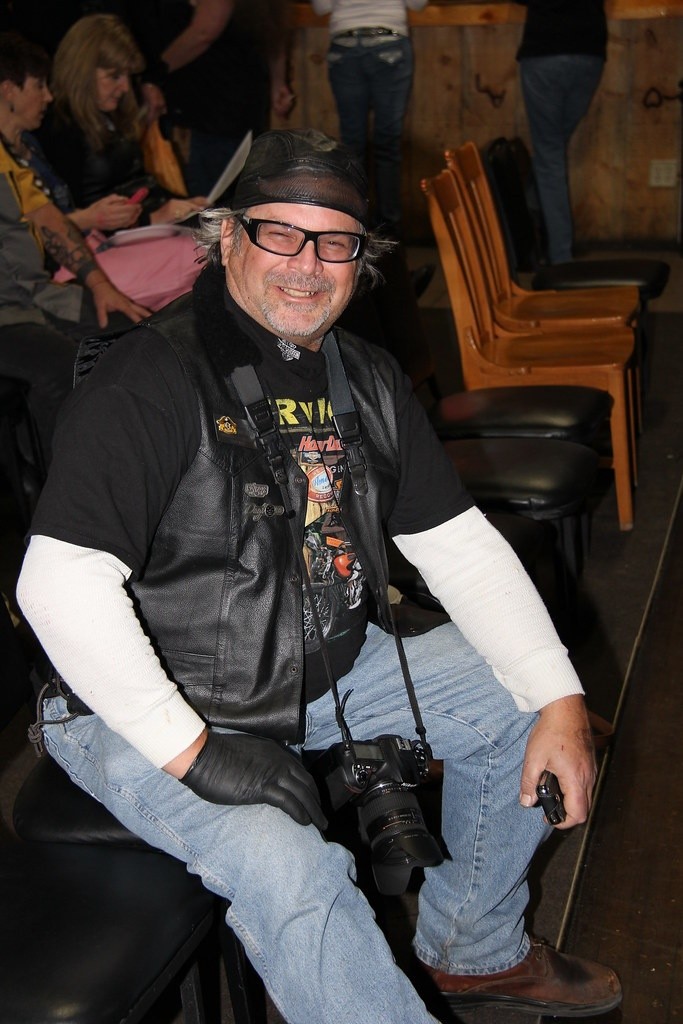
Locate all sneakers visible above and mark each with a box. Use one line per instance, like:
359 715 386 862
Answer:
416 934 623 1015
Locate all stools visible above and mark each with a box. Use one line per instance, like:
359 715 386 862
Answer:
1 836 269 1024
425 385 615 560
444 434 599 585
12 750 272 1024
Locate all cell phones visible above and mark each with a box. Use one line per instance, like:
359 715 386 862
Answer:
536 771 565 825
126 188 148 205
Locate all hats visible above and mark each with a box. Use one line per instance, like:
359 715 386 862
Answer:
239 124 373 223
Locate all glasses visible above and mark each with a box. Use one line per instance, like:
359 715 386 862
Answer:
236 213 368 264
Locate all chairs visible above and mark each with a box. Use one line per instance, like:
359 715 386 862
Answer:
420 168 639 533
480 133 671 386
443 141 645 435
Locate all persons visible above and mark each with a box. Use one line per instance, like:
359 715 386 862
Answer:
309 1 431 237
16 128 623 1024
33 13 209 231
510 2 610 268
1 35 154 475
143 2 284 174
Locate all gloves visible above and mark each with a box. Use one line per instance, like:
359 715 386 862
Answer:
179 731 328 829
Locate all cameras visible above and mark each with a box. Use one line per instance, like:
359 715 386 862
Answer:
321 733 443 894
